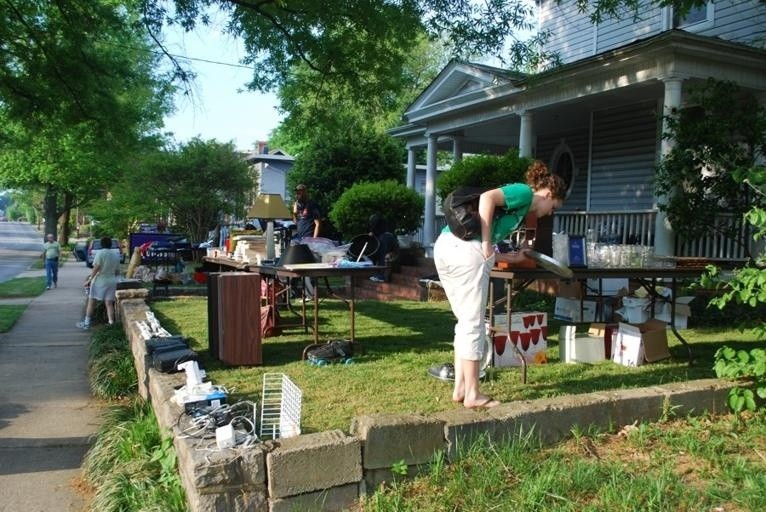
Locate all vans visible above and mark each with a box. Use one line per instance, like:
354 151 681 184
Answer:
71 238 123 268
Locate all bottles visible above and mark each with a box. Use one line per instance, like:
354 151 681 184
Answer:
586 228 596 269
551 234 571 268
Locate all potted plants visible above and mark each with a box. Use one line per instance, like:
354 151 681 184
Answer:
642 75 766 326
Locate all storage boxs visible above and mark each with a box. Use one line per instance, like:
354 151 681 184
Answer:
596 303 612 322
485 311 548 370
611 318 671 368
559 323 605 367
604 326 618 360
654 301 672 323
614 296 652 325
553 298 597 322
671 296 696 330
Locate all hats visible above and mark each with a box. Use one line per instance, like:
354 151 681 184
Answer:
294 184 306 191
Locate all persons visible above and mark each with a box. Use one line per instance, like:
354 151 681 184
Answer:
434 159 567 409
291 184 321 304
368 202 387 282
42 234 62 290
76 237 120 331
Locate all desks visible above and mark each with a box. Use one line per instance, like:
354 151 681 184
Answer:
483 266 721 384
208 271 264 366
202 256 388 361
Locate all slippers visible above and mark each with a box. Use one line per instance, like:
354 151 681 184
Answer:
428 362 455 381
470 398 501 409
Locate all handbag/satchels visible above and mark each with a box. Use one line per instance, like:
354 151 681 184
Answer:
444 187 489 240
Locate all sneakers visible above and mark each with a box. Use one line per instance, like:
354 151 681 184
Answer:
47 282 57 290
75 321 91 330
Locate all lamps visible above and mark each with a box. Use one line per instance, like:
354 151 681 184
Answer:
247 193 293 261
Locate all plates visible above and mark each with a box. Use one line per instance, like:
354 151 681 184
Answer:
524 250 575 279
426 366 487 382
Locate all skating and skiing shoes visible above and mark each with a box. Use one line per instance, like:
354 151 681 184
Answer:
306 338 353 367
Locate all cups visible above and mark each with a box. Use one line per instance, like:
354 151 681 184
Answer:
593 243 657 269
